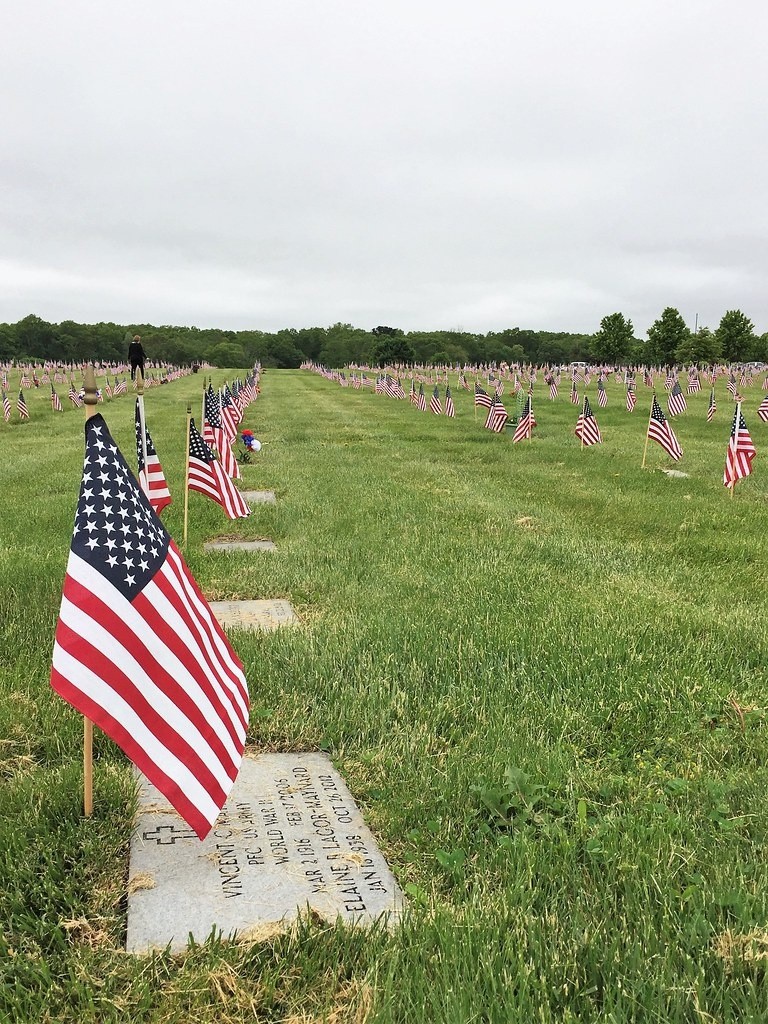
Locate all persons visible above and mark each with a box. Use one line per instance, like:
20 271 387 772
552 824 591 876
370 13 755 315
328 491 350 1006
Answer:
128 335 150 380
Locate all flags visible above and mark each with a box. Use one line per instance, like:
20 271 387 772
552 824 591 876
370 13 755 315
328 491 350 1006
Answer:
51 386 64 412
208 367 260 443
299 359 768 432
0 360 76 391
648 395 683 461
188 418 251 520
757 392 768 422
68 382 82 407
762 376 768 390
512 396 537 444
17 390 30 420
723 403 756 490
77 359 211 403
667 374 688 417
135 397 171 518
49 413 250 840
575 397 602 445
202 391 243 481
3 391 12 422
